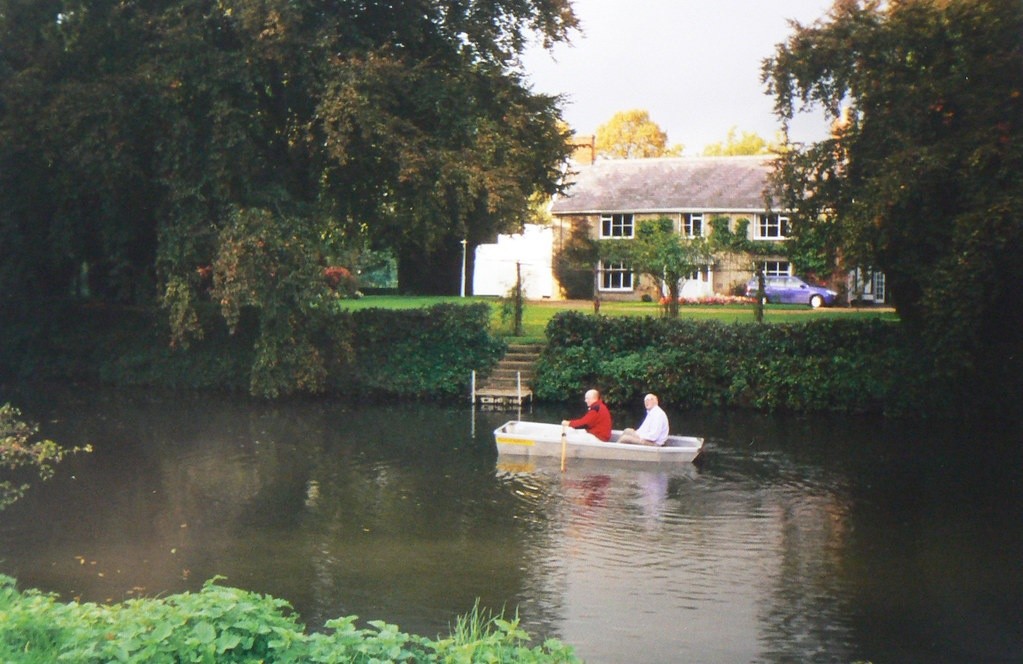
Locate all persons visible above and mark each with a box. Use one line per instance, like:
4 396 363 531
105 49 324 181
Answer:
562 389 612 443
620 394 669 444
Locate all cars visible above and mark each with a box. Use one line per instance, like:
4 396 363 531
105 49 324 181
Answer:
745 273 838 308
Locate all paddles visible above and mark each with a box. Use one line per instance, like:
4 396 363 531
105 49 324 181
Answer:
559 436 569 471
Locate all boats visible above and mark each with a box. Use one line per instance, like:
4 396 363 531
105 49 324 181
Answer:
493 417 706 463
